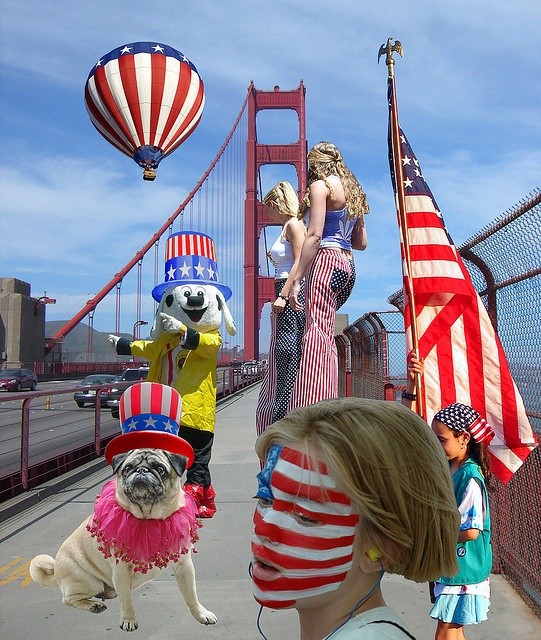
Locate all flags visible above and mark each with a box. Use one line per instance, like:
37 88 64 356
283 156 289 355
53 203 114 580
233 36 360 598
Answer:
379 67 540 486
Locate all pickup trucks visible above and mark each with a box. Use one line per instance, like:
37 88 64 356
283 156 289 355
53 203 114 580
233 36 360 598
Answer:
107 367 149 417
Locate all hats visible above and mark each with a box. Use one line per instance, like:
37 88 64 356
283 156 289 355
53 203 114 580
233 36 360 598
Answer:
104 383 195 471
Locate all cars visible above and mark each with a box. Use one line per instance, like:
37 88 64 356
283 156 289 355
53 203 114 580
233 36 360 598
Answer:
0 368 37 391
74 374 119 408
241 361 262 376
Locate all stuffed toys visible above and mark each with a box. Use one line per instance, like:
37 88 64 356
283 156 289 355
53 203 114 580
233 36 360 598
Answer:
106 230 237 520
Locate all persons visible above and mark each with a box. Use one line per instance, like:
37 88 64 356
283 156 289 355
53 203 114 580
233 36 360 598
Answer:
288 139 369 418
262 181 307 423
247 397 462 640
425 402 495 640
399 347 425 410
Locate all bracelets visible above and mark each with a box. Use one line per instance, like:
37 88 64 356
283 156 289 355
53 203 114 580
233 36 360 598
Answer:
402 391 417 401
278 292 289 301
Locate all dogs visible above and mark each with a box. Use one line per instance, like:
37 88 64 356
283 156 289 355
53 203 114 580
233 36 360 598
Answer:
29 447 218 631
